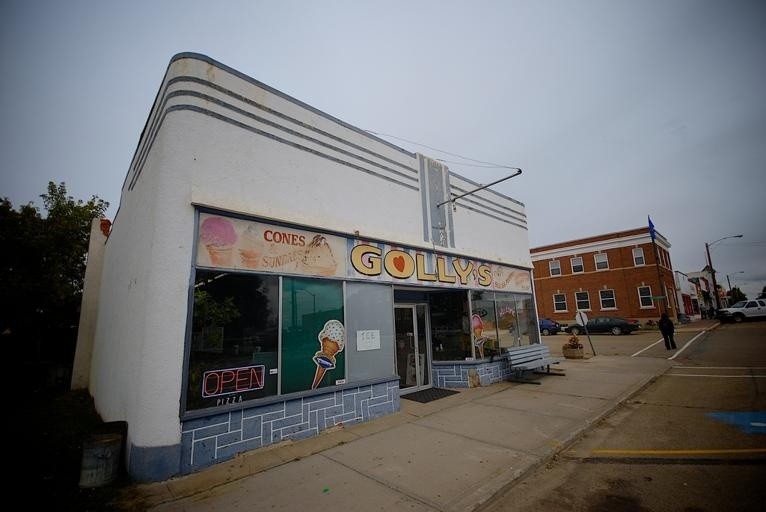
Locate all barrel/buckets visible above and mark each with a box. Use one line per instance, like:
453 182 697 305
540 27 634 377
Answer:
91 419 128 477
78 434 123 488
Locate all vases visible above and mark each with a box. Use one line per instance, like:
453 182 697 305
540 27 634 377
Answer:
564 349 584 359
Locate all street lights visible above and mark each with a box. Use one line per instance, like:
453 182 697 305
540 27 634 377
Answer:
295 286 316 313
705 233 744 310
726 270 744 290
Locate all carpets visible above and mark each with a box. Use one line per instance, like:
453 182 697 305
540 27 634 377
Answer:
400 387 461 404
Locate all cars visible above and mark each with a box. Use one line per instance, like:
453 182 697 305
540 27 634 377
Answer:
564 316 643 337
507 315 563 336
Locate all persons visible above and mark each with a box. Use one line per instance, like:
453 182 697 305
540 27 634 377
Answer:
659 314 676 350
700 304 706 319
727 304 731 308
714 304 721 319
708 305 714 319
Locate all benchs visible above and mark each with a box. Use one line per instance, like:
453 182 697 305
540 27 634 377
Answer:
504 343 566 385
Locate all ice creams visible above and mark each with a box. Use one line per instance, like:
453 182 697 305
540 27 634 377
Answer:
236 225 264 269
312 320 344 389
472 314 487 360
200 217 236 267
293 234 336 275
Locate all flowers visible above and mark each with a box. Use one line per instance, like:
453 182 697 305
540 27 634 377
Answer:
562 332 585 349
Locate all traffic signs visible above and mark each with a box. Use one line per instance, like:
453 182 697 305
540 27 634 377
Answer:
650 295 665 300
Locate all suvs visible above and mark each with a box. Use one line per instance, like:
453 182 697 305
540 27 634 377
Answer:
717 299 766 324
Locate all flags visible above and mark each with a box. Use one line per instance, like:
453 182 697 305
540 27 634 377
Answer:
648 217 656 240
